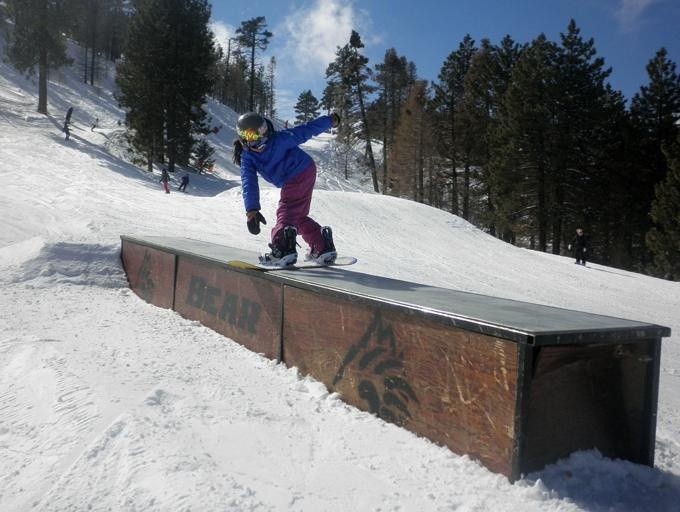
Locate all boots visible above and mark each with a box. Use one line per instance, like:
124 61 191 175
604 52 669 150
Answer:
303 226 335 262
259 225 298 265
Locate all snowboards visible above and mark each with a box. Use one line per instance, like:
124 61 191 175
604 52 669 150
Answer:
227 256 357 270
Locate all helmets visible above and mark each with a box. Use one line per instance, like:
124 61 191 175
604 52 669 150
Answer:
236 111 268 142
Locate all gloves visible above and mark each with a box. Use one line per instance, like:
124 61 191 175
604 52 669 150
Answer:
330 111 341 130
246 211 267 235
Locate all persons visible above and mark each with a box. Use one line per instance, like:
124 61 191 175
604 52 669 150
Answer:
178 174 190 192
567 228 589 266
63 123 72 141
90 118 99 131
232 111 340 268
158 169 170 193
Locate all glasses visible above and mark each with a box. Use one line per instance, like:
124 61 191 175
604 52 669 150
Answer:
247 134 270 149
